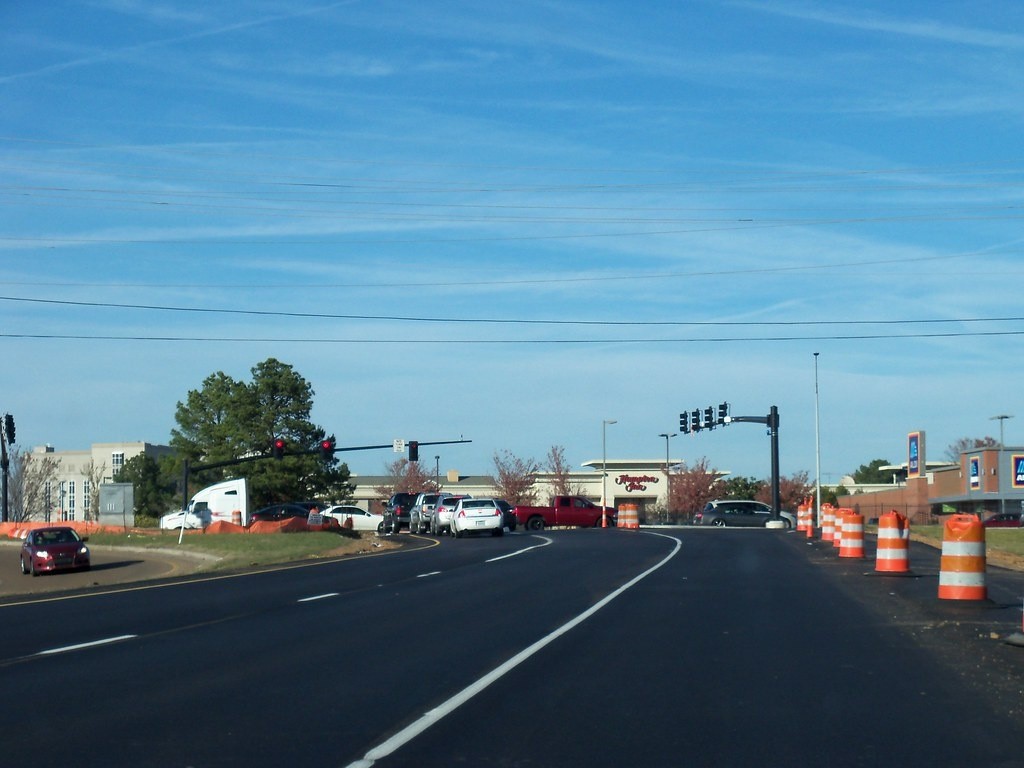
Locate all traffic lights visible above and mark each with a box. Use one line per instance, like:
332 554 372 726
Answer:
718 401 727 427
321 441 331 460
680 410 688 434
5 414 16 445
692 408 700 433
409 441 418 461
274 438 284 460
705 406 714 431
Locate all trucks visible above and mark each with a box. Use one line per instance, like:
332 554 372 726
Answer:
160 478 249 530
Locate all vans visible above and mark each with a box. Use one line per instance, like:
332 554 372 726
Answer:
703 499 797 529
982 513 1024 527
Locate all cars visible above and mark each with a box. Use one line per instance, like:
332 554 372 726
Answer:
494 500 517 531
693 503 792 529
449 498 504 538
21 526 91 577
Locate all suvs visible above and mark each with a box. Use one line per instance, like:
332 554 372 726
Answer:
249 490 472 536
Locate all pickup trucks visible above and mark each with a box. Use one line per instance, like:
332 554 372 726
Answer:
516 495 619 530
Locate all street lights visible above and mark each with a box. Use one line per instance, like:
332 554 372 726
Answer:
989 415 1015 513
658 433 677 523
602 420 617 527
